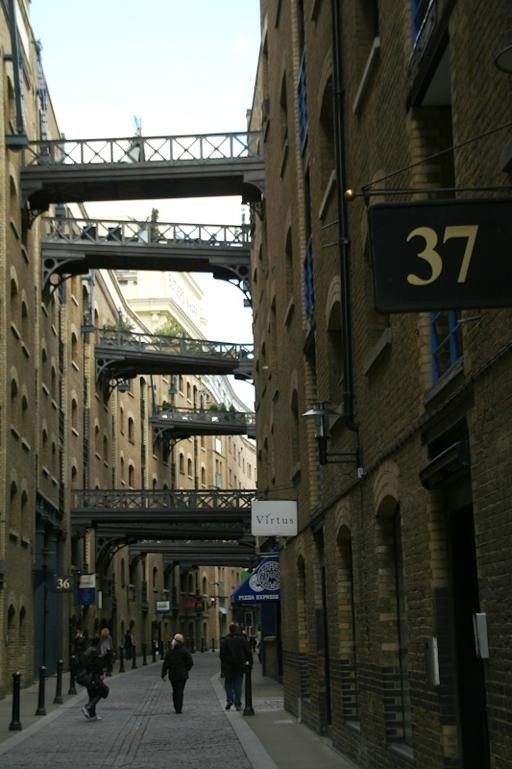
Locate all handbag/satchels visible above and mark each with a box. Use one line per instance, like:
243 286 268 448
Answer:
107 649 117 666
70 659 100 693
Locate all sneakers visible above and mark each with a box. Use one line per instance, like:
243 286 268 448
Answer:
81 707 90 719
236 706 243 710
89 716 102 721
226 700 233 709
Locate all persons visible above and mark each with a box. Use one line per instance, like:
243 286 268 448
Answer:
219 623 250 710
160 633 194 713
73 629 86 673
80 639 109 719
125 630 132 661
99 628 112 677
